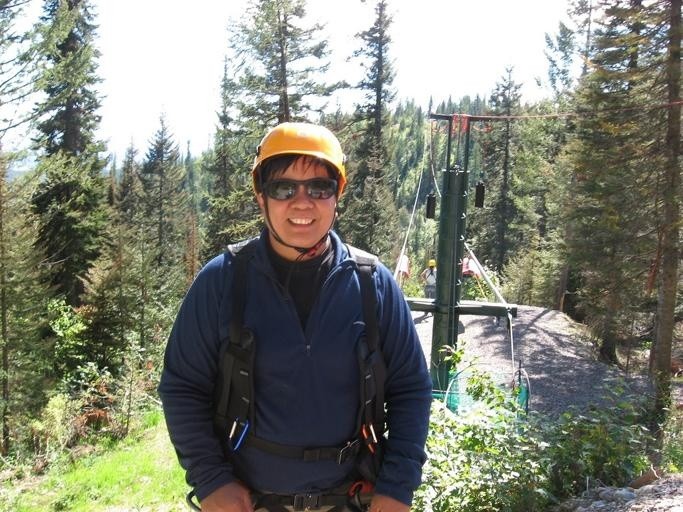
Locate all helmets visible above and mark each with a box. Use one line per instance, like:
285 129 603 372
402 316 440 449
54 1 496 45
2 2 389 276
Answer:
252 122 346 199
428 260 436 266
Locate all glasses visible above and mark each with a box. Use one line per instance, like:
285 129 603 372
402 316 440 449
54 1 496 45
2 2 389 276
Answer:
263 178 338 200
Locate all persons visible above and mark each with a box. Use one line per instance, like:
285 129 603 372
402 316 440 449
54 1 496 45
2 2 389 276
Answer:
156 121 433 511
419 259 436 315
